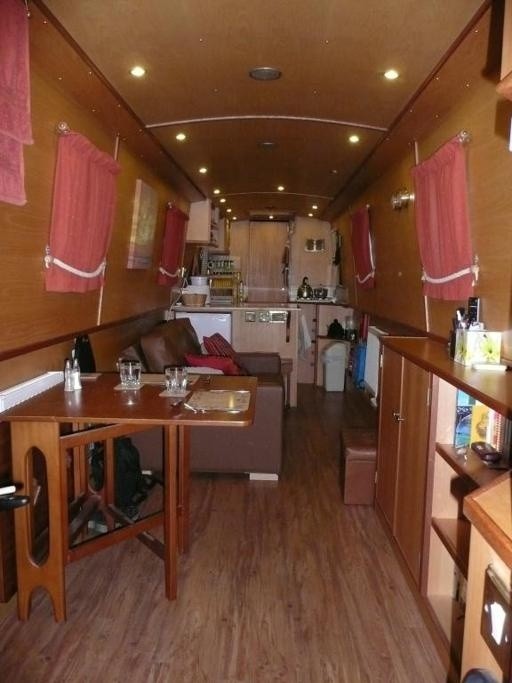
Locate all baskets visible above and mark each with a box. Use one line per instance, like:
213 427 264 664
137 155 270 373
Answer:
182 294 207 307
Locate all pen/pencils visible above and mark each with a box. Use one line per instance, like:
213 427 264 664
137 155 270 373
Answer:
183 403 198 414
452 308 469 329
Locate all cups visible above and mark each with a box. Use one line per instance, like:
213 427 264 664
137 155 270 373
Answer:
118 360 142 388
163 366 188 395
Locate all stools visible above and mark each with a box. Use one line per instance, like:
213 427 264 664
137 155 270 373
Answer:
340 425 378 506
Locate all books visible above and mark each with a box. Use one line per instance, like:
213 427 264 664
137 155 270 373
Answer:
454 389 512 457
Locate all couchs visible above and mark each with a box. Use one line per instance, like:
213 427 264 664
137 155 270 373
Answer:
120 316 285 482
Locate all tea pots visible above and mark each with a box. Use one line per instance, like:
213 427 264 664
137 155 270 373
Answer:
297 275 314 299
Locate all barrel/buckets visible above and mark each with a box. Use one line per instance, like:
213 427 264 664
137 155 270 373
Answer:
188 274 209 285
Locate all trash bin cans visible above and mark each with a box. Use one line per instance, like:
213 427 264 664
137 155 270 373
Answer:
322 341 347 392
351 343 366 390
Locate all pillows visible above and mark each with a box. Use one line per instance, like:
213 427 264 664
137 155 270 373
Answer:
180 331 251 376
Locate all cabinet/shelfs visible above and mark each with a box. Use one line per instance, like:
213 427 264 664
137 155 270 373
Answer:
378 345 433 588
185 197 220 247
422 371 512 683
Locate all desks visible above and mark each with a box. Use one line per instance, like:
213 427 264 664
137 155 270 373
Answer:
0 369 259 626
